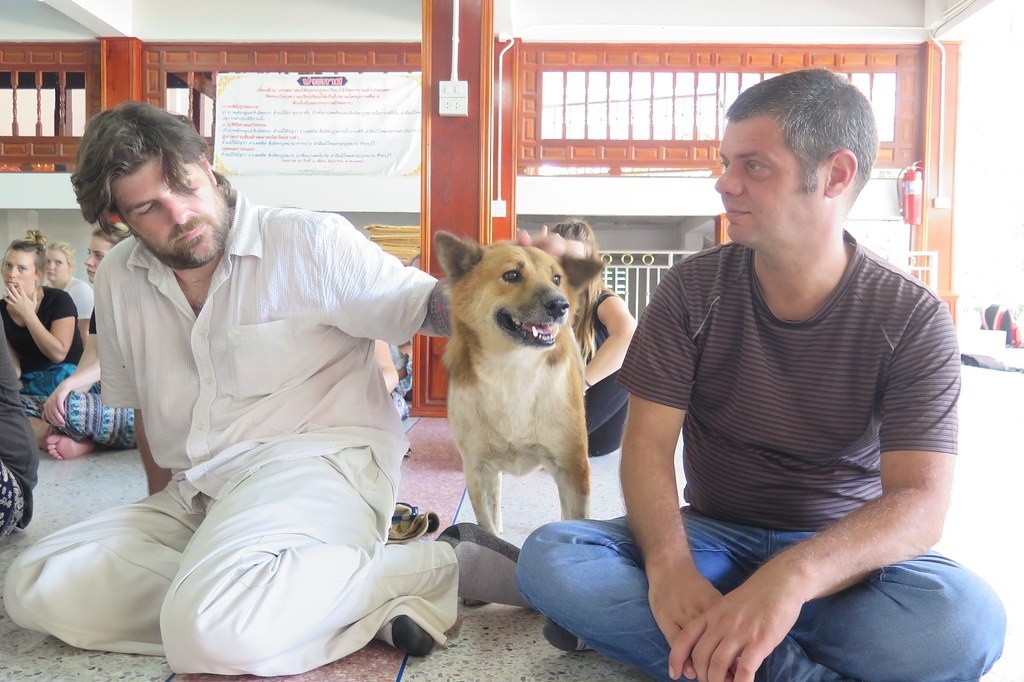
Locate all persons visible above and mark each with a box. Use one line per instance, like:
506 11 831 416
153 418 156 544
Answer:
4 102 582 675
514 69 1005 681
545 217 637 458
0 222 139 541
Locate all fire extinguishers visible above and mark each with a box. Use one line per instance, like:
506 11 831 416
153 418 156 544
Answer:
896 160 924 226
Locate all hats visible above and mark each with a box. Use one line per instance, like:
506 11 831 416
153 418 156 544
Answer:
386 504 441 543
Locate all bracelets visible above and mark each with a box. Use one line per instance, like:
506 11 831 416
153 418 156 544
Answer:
584 380 592 388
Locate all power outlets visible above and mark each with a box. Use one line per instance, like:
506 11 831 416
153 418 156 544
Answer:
439 98 469 117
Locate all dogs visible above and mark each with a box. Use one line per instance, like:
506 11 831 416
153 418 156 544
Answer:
433 230 607 538
